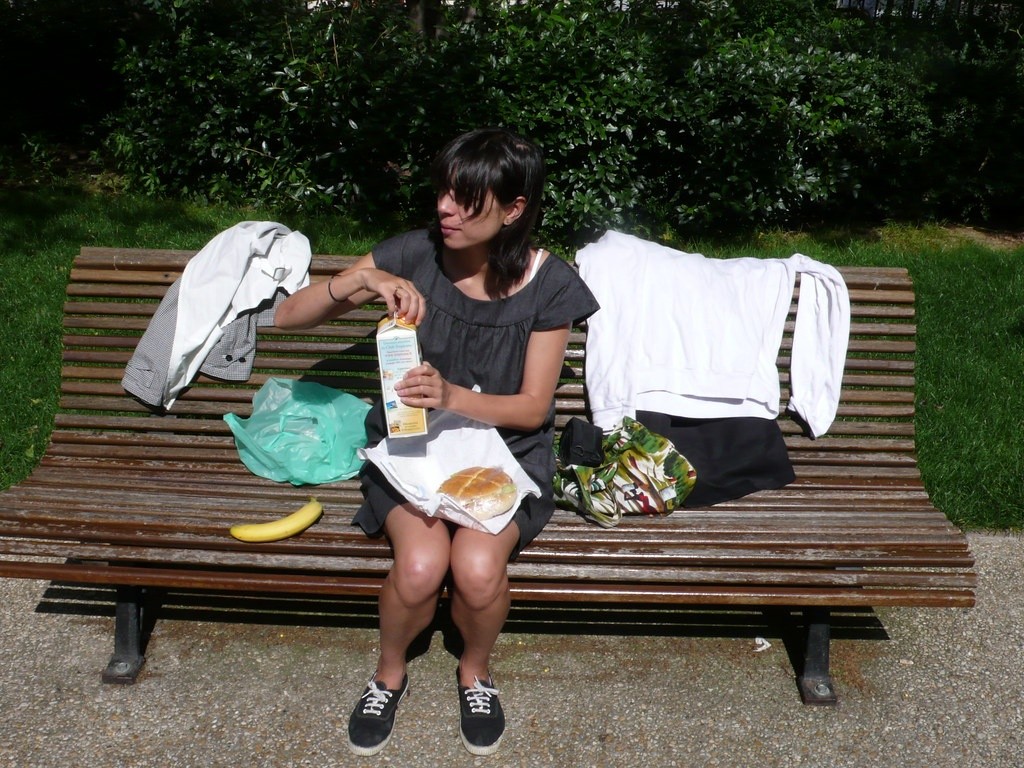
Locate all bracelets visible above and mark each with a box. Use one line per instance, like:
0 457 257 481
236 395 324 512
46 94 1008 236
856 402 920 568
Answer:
328 274 348 302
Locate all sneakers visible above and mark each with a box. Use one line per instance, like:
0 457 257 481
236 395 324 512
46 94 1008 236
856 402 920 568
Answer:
347 670 409 756
455 665 506 755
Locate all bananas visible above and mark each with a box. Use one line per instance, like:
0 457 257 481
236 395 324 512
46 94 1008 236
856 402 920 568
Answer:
229 495 322 542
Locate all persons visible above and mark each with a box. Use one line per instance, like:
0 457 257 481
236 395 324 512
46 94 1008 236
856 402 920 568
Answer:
275 127 603 758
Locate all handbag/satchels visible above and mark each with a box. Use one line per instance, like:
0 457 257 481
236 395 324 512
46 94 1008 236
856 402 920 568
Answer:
552 415 699 529
222 376 373 486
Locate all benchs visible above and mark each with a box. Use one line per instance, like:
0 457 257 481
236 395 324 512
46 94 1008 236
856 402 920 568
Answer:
0 245 981 709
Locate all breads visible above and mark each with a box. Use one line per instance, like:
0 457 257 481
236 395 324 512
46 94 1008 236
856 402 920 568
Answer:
436 466 517 520
